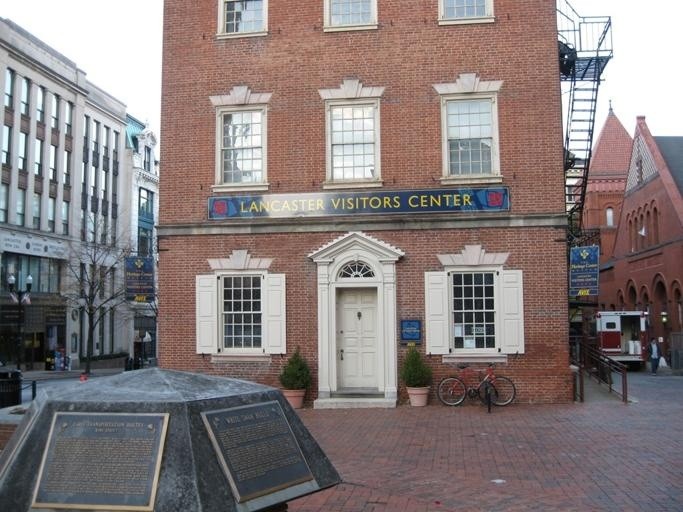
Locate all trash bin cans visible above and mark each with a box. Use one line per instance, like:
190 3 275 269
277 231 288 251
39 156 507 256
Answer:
0 368 22 409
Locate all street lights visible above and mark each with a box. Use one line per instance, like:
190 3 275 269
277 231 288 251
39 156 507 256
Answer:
138 326 147 368
6 272 34 368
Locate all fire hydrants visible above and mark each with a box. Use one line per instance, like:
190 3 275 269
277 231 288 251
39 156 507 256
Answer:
79 372 89 381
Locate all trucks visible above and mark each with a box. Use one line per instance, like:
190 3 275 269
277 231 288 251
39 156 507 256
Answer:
586 309 650 372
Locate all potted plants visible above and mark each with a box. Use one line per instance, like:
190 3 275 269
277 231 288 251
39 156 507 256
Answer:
279 346 313 409
400 344 434 407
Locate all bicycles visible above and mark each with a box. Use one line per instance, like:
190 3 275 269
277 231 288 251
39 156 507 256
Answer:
437 362 516 408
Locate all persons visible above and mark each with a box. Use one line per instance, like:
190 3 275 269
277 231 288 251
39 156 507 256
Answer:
128 358 134 371
647 337 660 376
123 357 129 372
77 370 89 383
